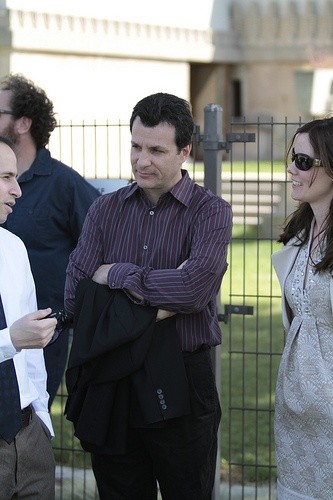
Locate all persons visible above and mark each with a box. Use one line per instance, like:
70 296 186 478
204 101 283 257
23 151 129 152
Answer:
0 73 102 411
64 92 234 500
270 117 333 500
0 135 56 500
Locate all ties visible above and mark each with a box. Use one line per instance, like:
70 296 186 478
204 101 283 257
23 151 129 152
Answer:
0 292 25 445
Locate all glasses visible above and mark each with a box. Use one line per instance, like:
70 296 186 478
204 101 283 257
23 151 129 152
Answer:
291 147 323 171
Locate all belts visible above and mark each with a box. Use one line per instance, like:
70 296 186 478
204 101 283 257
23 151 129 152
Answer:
19 407 37 428
